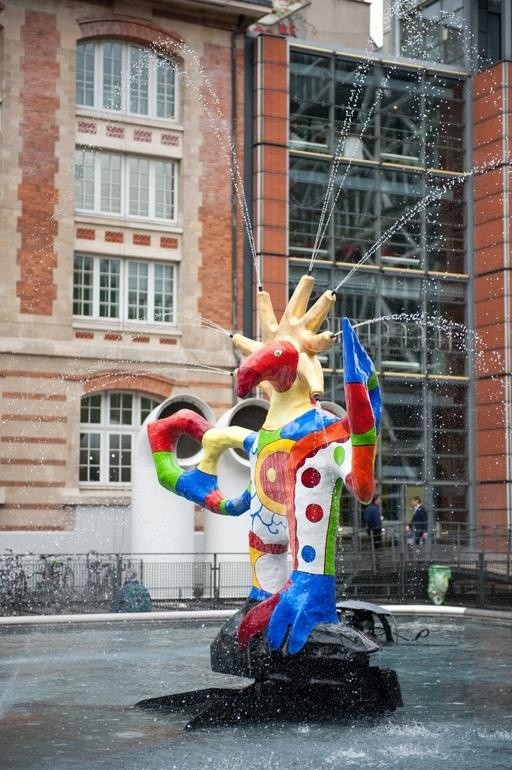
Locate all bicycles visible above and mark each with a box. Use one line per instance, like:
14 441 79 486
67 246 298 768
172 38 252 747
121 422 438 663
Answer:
1 548 138 616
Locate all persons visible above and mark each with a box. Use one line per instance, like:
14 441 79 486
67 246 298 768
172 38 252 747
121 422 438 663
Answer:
406 497 427 544
364 495 382 549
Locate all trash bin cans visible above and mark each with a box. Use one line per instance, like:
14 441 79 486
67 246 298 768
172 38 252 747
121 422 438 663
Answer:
427 565 451 605
